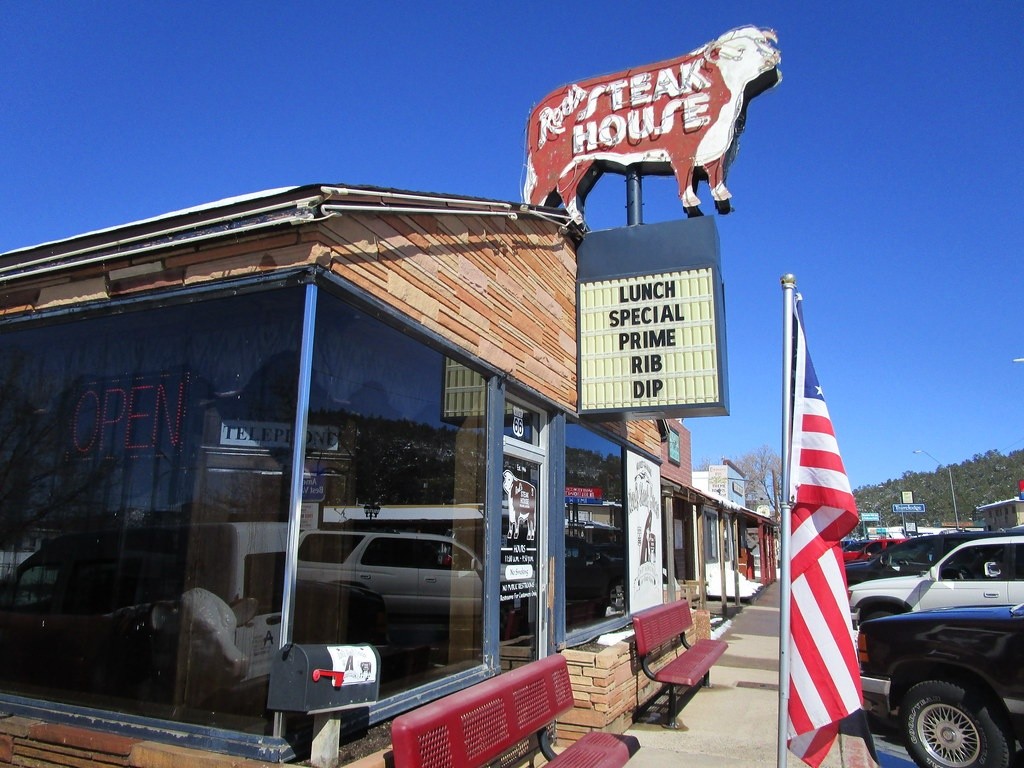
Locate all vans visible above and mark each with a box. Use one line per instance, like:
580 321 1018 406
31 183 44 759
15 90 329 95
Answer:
0 519 289 695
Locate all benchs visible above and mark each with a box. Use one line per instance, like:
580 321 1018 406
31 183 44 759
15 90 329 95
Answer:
631 599 729 731
391 654 641 767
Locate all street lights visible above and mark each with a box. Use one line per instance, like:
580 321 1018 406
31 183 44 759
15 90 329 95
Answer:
913 450 959 529
878 485 907 536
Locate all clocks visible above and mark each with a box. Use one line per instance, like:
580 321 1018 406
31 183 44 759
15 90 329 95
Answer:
667 425 680 466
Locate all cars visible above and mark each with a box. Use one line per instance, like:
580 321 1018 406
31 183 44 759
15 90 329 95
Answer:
565 534 671 610
842 538 911 564
844 539 875 551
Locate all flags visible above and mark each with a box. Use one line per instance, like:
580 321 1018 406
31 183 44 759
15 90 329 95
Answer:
786 299 863 768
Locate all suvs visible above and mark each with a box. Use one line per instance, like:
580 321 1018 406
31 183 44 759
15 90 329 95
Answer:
858 605 1024 768
846 536 1024 621
844 528 1022 587
297 529 534 644
